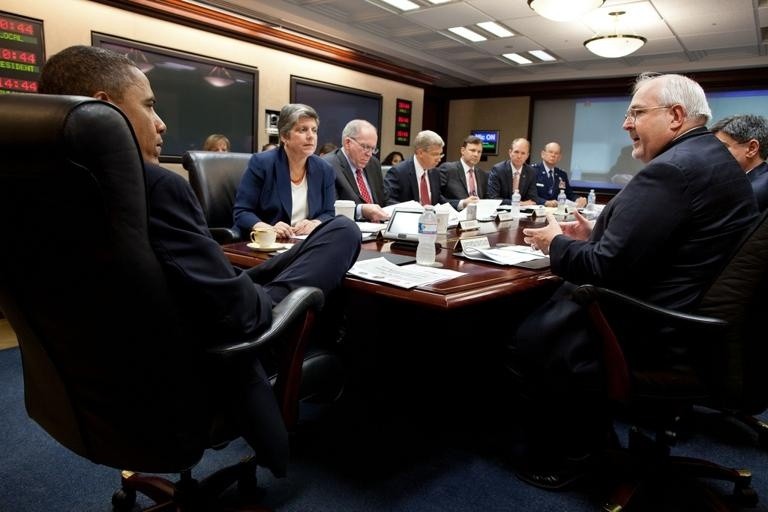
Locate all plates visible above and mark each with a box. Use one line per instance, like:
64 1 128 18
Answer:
245 242 284 252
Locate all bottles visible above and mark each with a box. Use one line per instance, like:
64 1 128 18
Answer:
509 189 522 221
556 190 567 217
415 204 439 266
587 188 596 213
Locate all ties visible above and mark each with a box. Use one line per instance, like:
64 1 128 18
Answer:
513 172 519 191
356 168 372 203
421 172 431 204
469 169 477 196
549 170 553 185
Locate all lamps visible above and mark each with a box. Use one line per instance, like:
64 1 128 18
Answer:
526 0 607 24
580 10 647 58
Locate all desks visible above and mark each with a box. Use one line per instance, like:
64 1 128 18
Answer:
212 219 604 489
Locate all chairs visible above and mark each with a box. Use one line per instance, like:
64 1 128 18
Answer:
663 404 767 452
0 87 348 512
177 147 260 248
496 205 767 512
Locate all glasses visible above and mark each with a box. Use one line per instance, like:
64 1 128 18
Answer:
351 137 379 155
624 106 670 123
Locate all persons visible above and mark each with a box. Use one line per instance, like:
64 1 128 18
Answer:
36 44 365 407
319 143 338 158
608 141 651 189
262 143 278 153
486 137 539 207
381 151 404 165
437 135 487 212
703 114 767 255
319 118 390 224
516 71 762 489
382 129 480 212
230 103 337 239
528 140 587 209
200 132 231 152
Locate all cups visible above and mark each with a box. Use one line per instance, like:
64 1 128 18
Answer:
437 212 450 236
333 200 357 221
249 228 278 247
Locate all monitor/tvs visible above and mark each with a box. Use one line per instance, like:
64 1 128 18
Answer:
471 129 500 156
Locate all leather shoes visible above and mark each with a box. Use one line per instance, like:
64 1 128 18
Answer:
514 461 572 489
